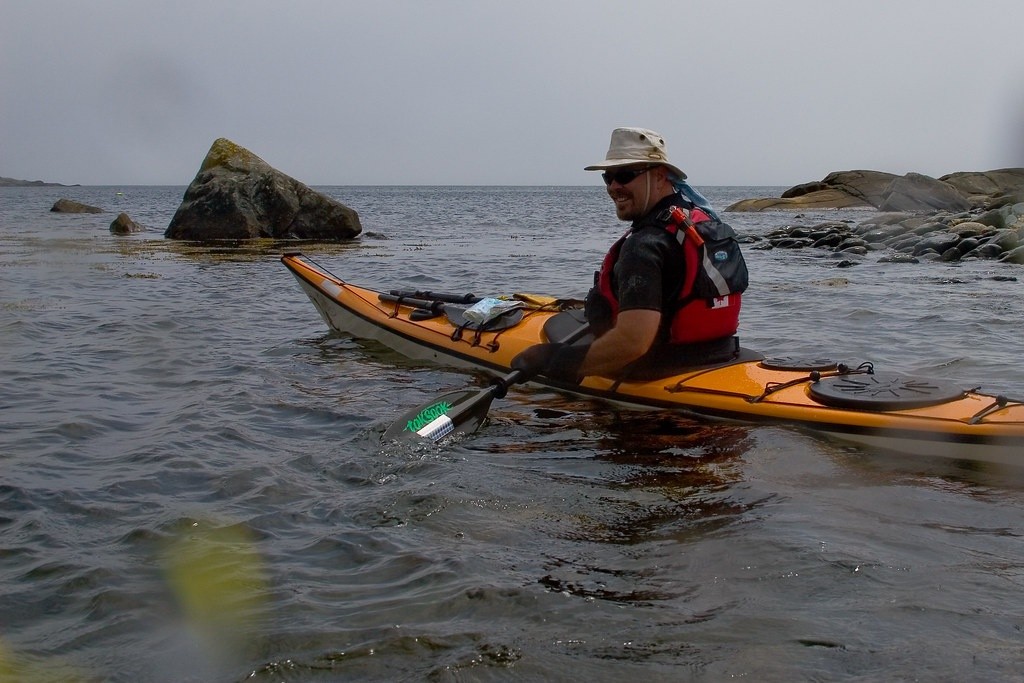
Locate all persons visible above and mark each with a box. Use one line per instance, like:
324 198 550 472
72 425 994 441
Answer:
510 128 749 381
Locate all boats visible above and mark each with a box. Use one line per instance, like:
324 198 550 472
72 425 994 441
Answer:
282 252 1024 463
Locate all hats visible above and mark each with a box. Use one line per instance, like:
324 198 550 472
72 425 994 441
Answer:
584 127 688 179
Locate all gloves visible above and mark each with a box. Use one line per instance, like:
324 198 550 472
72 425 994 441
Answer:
510 343 592 387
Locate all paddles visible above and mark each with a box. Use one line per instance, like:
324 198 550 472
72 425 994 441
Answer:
380 322 591 444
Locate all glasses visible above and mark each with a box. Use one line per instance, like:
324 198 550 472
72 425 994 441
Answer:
601 163 662 186
